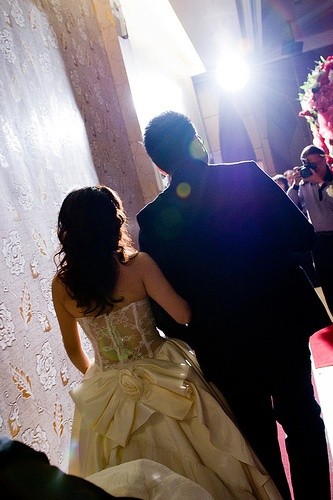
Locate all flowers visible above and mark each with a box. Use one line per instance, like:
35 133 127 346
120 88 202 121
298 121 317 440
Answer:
295 54 333 170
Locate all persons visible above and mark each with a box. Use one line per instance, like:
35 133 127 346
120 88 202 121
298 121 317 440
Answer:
51 186 281 500
275 148 333 316
136 111 333 500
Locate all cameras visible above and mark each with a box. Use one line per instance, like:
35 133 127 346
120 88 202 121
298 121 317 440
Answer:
298 158 317 178
